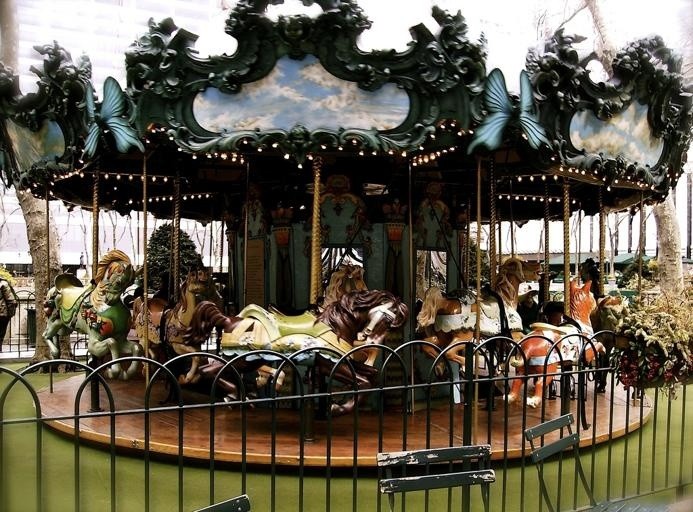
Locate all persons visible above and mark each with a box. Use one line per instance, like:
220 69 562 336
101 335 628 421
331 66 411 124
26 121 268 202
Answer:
0 277 18 353
41 249 619 415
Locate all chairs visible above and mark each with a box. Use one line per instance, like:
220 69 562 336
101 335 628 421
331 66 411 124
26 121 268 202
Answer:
376 412 670 511
